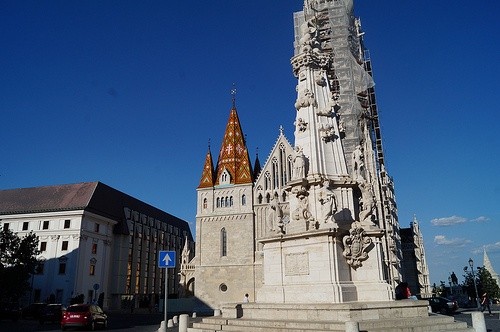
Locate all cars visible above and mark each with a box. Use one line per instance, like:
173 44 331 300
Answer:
60 303 109 332
32 302 62 326
421 297 458 316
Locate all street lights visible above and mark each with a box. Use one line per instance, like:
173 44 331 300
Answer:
468 258 482 312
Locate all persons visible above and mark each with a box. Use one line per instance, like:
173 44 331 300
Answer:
318 181 337 222
243 294 249 303
395 282 418 300
359 183 378 222
296 186 313 221
353 145 363 179
124 297 127 306
292 147 305 179
79 294 84 304
98 293 104 308
481 293 494 316
149 303 152 311
130 298 135 313
